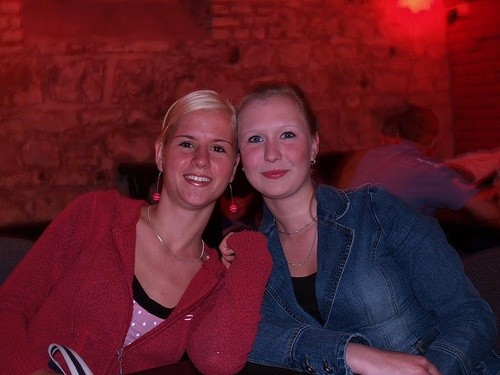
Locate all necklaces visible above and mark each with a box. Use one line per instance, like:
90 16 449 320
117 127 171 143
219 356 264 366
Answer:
146 204 204 263
277 216 317 268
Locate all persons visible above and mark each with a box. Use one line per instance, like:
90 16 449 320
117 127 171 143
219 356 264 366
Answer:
0 90 273 374
237 82 495 375
339 104 500 221
